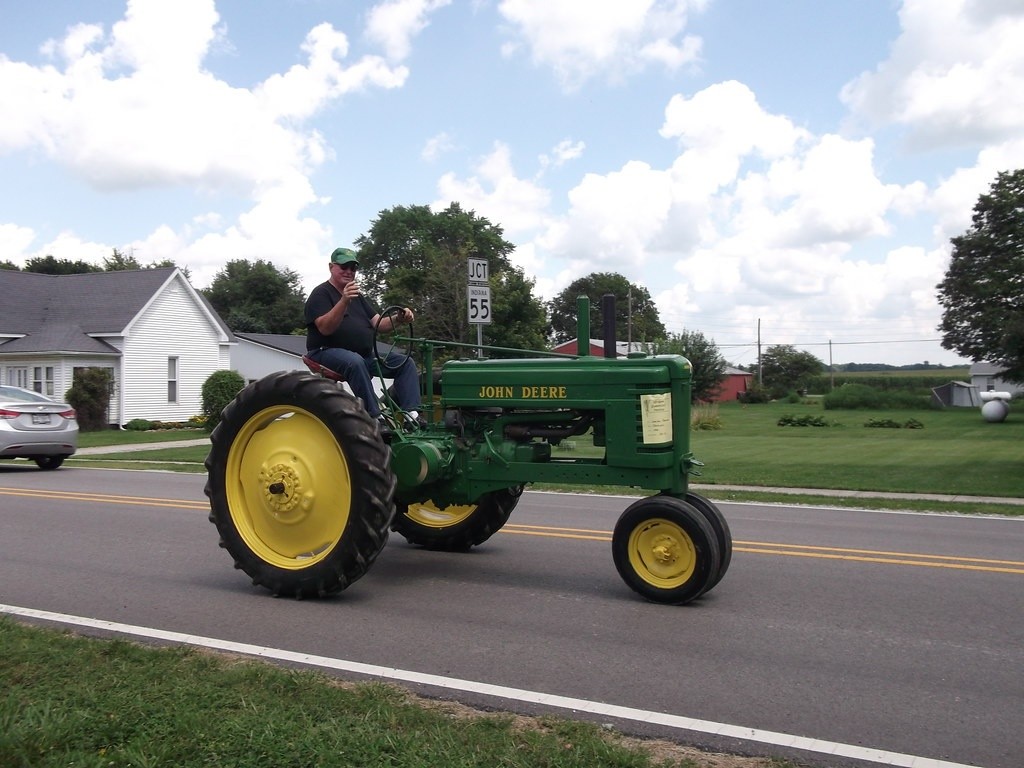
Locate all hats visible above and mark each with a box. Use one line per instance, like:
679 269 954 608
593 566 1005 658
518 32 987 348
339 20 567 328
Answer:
331 248 359 265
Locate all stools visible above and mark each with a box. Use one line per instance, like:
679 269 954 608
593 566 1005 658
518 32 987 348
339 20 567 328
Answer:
303 354 346 384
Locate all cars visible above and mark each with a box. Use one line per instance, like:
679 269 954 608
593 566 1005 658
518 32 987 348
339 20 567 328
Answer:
0 385 80 471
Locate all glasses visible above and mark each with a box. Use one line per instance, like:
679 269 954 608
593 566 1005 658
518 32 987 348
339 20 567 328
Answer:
332 262 357 272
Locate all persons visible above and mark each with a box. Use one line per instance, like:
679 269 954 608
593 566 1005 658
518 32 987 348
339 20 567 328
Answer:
306 248 427 447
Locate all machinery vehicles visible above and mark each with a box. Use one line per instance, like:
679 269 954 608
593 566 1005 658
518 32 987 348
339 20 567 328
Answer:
203 293 733 606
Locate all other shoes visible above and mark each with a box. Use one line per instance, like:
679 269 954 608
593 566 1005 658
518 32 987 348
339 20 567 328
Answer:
378 418 392 435
403 416 428 431
